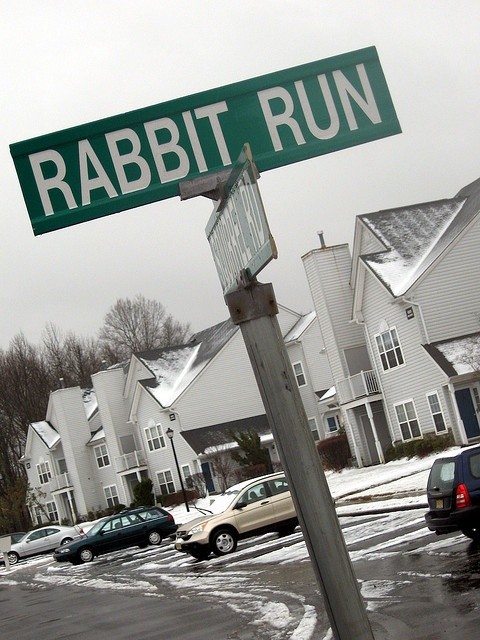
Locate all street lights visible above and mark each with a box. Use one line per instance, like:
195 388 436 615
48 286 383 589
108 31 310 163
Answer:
166 428 190 513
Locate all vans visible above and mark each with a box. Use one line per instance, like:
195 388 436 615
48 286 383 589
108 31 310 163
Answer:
0 531 27 544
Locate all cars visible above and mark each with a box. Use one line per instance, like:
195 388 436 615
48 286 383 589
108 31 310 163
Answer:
0 524 85 565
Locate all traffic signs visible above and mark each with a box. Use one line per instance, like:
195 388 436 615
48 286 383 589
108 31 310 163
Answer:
8 44 403 237
203 140 278 301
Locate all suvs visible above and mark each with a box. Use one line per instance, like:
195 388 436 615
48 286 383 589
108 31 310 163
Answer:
173 470 299 560
424 443 480 540
53 506 178 563
